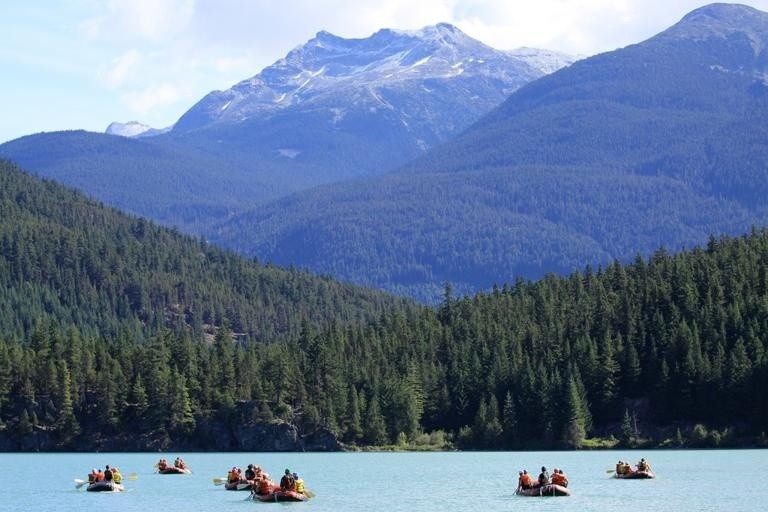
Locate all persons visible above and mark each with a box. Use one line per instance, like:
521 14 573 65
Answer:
517 467 569 490
154 455 187 469
84 464 122 484
227 461 307 494
613 457 652 473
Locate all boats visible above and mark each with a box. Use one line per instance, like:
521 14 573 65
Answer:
224 479 258 491
517 478 571 497
86 481 123 492
254 489 306 502
158 467 186 474
615 469 655 479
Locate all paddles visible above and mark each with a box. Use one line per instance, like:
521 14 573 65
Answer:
213 477 228 482
185 466 192 475
75 481 90 489
73 478 88 483
606 469 616 473
215 481 228 485
123 476 138 481
121 471 137 476
302 488 316 499
647 466 656 478
